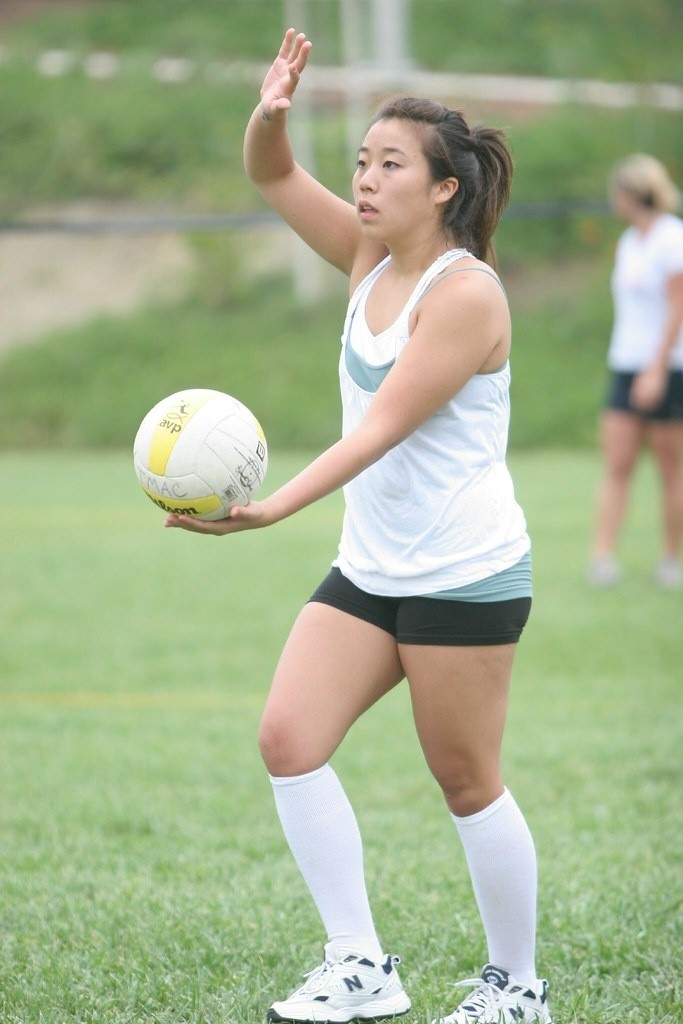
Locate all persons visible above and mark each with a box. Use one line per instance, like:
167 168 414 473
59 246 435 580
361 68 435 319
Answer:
165 25 550 1024
588 154 683 592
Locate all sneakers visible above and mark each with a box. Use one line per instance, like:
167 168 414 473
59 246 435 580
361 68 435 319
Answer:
268 942 411 1024
432 963 555 1024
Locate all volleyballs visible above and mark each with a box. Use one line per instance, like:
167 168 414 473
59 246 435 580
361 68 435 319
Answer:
138 388 271 521
